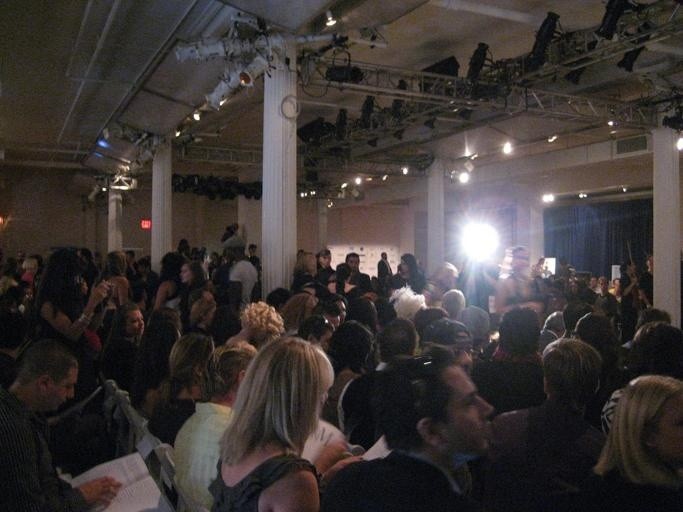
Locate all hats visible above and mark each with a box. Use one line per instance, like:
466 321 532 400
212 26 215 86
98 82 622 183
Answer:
421 318 473 347
569 313 609 339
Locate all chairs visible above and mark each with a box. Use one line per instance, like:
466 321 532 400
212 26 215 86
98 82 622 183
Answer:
104 377 210 511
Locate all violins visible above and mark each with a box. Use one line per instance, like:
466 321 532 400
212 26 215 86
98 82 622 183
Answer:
631 271 644 311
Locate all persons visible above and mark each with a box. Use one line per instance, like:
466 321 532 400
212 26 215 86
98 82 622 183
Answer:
0 221 682 512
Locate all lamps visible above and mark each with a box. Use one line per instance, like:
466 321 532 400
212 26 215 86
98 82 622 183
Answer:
83 0 683 200
